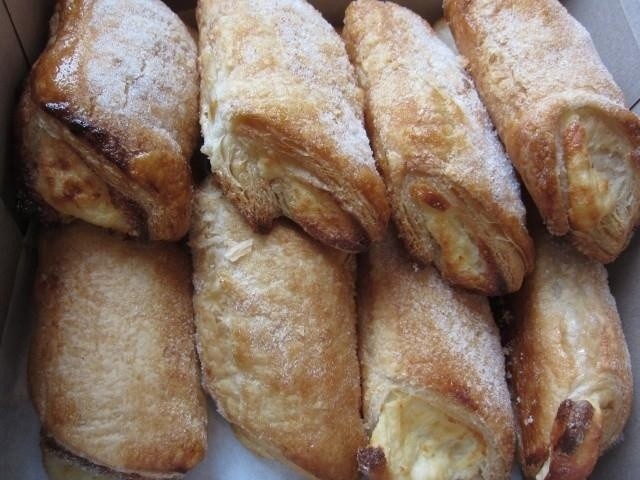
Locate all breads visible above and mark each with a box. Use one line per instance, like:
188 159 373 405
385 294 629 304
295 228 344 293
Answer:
441 0 640 267
492 212 633 479
26 225 207 480
15 0 196 243
188 173 369 480
357 243 514 479
341 0 533 295
195 1 390 253
